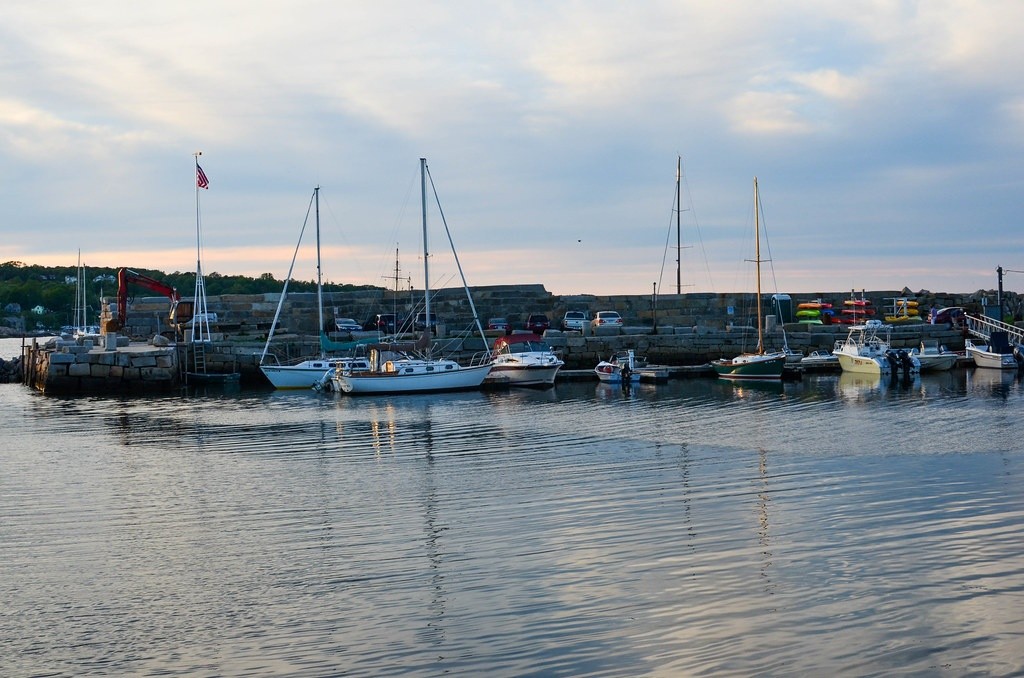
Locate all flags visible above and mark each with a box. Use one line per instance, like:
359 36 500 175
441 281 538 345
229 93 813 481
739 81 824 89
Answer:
197 163 209 189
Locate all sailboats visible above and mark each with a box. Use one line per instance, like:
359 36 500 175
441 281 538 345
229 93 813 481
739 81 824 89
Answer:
711 175 788 379
332 157 494 395
258 185 375 391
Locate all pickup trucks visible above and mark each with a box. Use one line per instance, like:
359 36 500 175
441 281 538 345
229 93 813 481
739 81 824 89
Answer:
560 311 586 333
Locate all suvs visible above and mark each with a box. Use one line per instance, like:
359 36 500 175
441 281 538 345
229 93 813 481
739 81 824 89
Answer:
525 312 551 334
412 312 438 332
373 314 400 333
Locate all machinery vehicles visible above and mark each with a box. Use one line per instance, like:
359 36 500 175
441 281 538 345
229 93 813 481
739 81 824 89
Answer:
103 267 240 349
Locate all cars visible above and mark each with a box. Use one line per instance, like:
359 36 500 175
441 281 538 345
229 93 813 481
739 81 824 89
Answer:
324 317 362 336
590 310 623 334
485 318 511 335
928 306 981 325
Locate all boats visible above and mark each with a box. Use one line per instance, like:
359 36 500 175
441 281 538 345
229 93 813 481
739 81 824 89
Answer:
830 299 921 376
595 356 641 380
966 333 1019 369
780 349 804 367
901 340 958 370
801 348 838 363
472 341 565 386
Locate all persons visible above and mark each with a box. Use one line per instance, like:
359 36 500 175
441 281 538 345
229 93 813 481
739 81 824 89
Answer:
973 311 980 319
930 304 937 324
898 349 911 373
885 350 898 373
951 309 960 323
